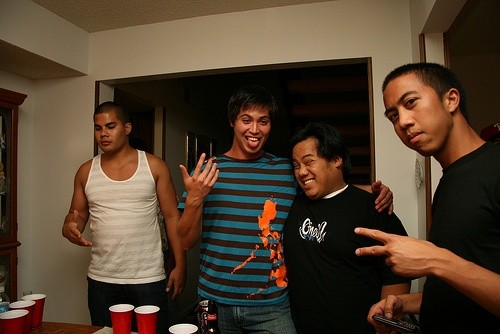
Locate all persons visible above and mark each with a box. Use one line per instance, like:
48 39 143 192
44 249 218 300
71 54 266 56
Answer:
176 85 412 334
353 62 500 334
61 100 188 334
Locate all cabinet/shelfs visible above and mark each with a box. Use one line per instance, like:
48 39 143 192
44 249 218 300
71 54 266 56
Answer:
0 87 28 303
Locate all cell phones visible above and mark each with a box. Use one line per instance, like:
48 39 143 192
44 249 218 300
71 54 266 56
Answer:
372 313 420 334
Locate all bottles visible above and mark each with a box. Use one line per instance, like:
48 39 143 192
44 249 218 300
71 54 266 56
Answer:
0 283 11 313
207 300 218 334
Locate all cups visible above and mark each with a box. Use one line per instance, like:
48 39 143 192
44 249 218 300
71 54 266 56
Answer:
169 324 198 334
134 305 160 334
109 304 134 334
20 294 47 327
0 310 29 334
9 301 36 334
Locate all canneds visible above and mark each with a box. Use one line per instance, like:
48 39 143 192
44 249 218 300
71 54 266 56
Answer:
196 300 219 334
